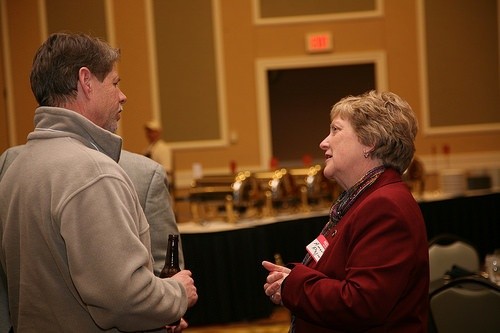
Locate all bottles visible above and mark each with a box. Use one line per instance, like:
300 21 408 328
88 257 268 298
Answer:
158 235 181 325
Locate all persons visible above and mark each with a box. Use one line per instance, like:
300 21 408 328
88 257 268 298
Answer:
262 91 431 333
0 32 199 333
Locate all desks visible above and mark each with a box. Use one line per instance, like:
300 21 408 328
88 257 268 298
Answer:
175 186 500 326
430 275 500 291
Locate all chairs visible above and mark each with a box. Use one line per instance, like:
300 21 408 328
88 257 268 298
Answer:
429 239 479 282
430 280 500 333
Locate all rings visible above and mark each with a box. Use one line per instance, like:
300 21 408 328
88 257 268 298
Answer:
270 294 275 301
